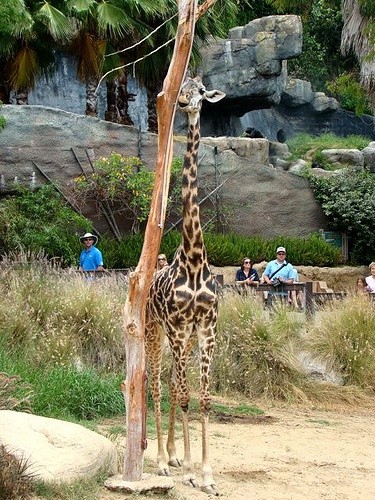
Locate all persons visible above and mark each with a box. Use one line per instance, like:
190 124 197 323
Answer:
291 268 306 309
234 256 260 283
78 233 103 272
155 253 168 270
356 276 368 288
257 270 269 299
366 262 375 294
288 290 292 303
262 246 295 306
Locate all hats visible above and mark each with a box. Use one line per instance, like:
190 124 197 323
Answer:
80 233 98 246
276 247 286 253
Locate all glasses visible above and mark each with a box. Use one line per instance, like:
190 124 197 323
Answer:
243 261 252 264
158 258 165 260
84 239 93 241
278 253 285 255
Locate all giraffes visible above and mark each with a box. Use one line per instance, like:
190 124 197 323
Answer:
144 72 227 497
